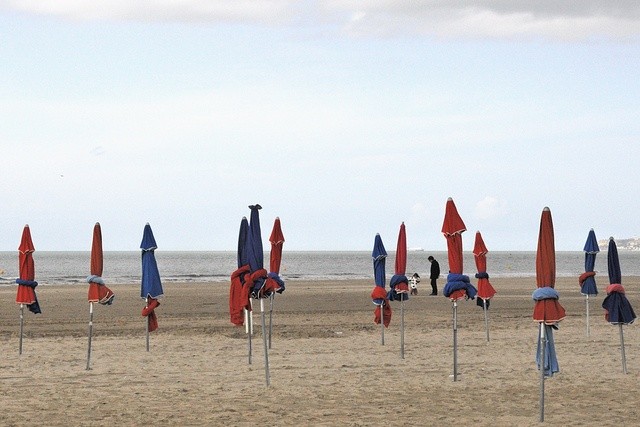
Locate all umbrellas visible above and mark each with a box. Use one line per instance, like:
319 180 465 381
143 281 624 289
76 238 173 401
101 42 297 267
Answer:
579 228 601 340
264 217 285 349
441 197 478 382
16 224 41 355
471 231 497 342
140 223 163 351
387 221 410 359
86 222 114 370
229 216 253 364
249 204 270 387
370 232 392 345
531 206 568 422
602 236 637 374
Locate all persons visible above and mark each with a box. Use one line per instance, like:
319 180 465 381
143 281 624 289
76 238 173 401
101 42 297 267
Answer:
408 273 420 295
428 256 440 296
